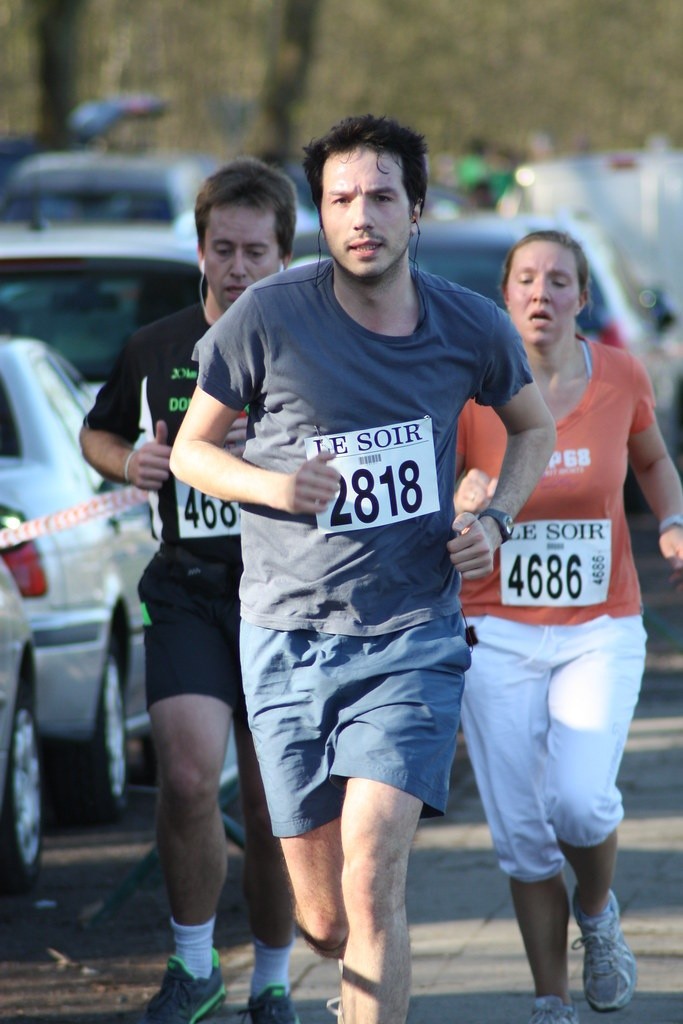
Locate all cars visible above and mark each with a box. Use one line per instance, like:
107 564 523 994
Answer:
0 556 48 898
0 339 160 823
0 152 682 517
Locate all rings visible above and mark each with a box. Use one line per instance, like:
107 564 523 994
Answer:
469 492 476 501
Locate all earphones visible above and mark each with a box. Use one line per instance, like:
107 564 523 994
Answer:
201 258 207 275
412 215 416 224
279 262 285 274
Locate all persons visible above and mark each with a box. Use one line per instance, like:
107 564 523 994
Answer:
169 113 558 1024
79 158 299 1024
453 231 683 1024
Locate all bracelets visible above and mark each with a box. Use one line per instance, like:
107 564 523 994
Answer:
124 451 136 483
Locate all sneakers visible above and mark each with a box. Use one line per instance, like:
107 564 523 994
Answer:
238 983 300 1024
571 882 637 1012
530 995 579 1024
138 947 226 1024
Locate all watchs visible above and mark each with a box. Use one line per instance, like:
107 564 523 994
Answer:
478 508 514 544
658 513 683 534
